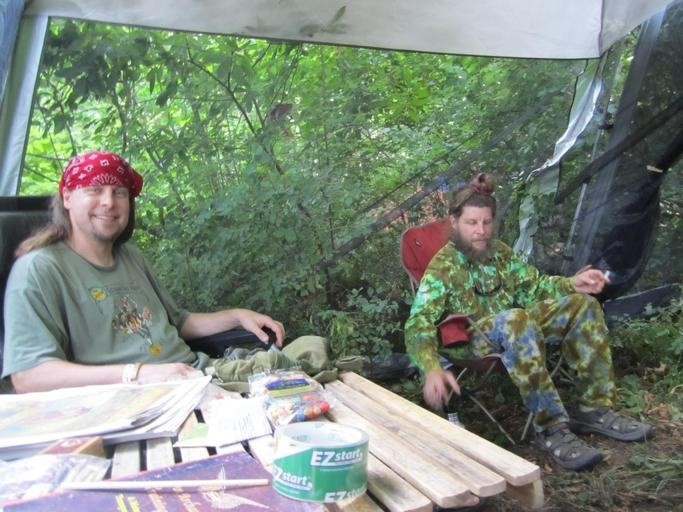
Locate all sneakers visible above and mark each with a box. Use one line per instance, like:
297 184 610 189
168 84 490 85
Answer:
534 422 603 470
569 400 652 441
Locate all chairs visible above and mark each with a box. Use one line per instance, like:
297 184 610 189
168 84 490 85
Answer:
0 196 277 394
398 216 573 444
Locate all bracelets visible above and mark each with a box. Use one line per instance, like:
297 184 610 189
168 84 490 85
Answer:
122 363 135 384
130 362 142 383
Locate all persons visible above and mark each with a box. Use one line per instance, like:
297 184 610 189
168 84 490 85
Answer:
0 150 333 395
402 170 652 470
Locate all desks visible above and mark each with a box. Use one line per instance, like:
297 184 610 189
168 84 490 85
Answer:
0 372 545 512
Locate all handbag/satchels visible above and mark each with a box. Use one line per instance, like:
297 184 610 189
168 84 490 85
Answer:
217 335 364 392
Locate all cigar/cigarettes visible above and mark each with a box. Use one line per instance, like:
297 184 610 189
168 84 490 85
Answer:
598 270 612 280
